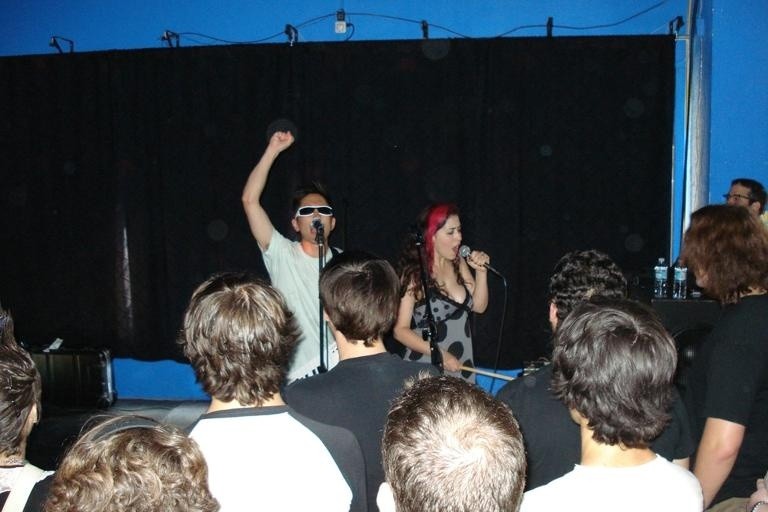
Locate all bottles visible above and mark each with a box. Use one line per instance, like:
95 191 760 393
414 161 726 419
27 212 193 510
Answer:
652 256 669 301
671 257 688 299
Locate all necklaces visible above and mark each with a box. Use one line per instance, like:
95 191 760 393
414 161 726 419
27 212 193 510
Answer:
1 457 24 463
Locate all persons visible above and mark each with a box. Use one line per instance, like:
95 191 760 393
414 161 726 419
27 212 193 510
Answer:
174 134 768 512
43 414 221 511
0 313 55 512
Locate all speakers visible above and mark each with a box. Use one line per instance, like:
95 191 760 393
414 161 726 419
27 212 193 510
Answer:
640 284 722 375
14 341 117 413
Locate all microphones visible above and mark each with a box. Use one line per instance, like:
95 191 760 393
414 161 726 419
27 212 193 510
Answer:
311 217 324 235
459 245 503 278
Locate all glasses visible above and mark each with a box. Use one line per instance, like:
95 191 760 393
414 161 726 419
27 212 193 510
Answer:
294 206 333 219
